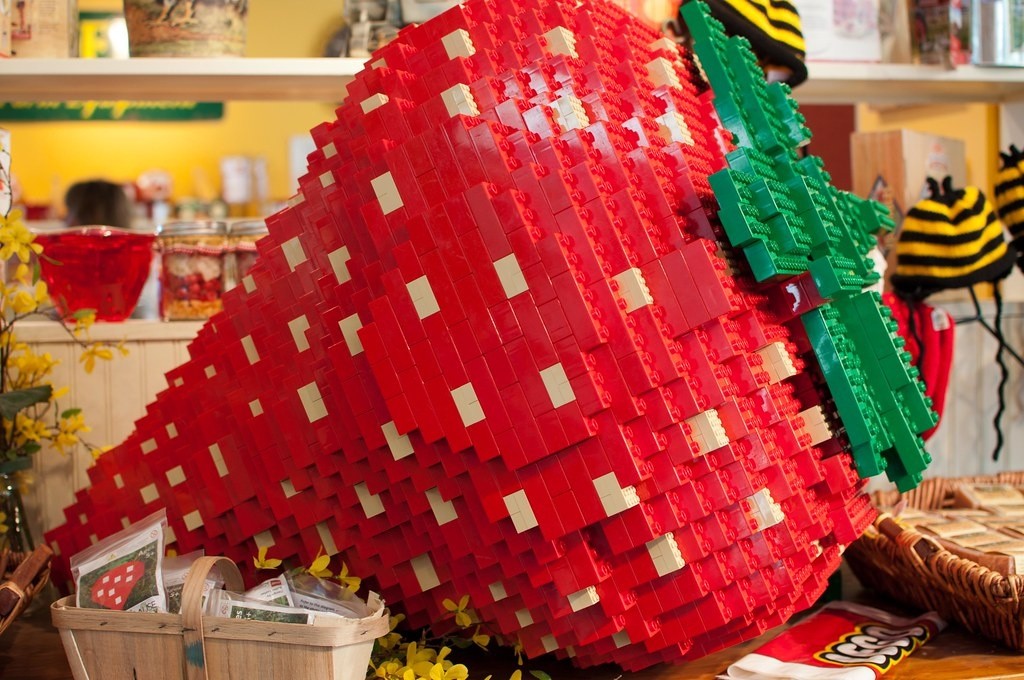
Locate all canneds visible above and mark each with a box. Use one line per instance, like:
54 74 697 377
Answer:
156 219 278 321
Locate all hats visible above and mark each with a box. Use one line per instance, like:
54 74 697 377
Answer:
892 175 1018 303
677 1 805 93
994 144 1024 273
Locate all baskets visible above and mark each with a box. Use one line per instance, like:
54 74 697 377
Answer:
841 472 1024 656
51 555 392 679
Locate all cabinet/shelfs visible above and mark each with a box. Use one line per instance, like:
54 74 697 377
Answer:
1 0 1024 556
